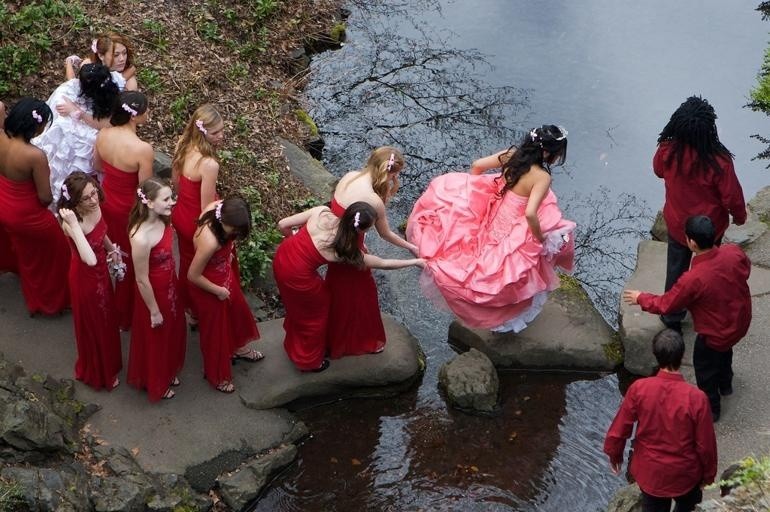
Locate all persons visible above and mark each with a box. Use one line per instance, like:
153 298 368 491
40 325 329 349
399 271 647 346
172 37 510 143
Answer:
1 33 262 402
604 329 717 512
623 215 751 423
325 147 419 360
273 202 428 372
653 95 747 335
405 125 576 334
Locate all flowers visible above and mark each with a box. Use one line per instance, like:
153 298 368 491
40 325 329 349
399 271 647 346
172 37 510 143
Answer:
105 245 129 286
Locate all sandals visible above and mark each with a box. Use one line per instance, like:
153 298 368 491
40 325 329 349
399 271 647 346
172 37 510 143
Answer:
216 379 236 393
233 348 265 362
162 378 180 399
